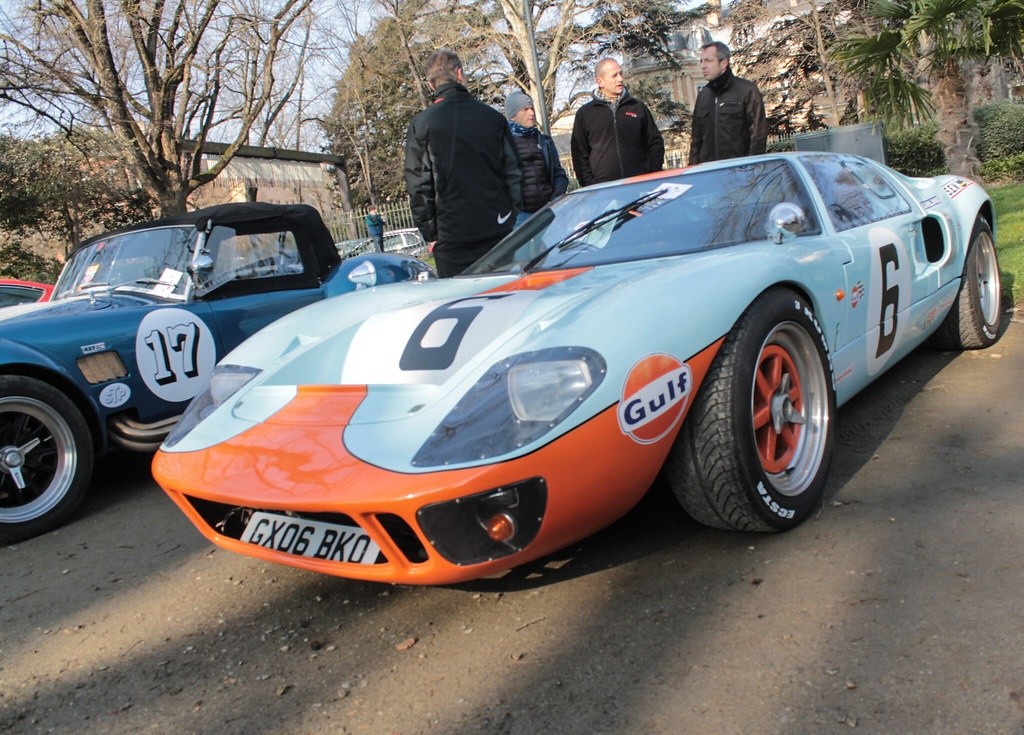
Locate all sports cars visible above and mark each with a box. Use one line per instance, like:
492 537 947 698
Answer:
150 151 1006 587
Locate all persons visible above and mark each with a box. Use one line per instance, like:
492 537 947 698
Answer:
688 42 768 165
366 205 387 252
571 58 665 187
404 51 569 279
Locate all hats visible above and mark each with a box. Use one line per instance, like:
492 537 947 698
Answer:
367 205 376 212
505 92 533 118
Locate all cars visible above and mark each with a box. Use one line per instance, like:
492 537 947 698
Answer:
0 201 439 551
334 227 427 261
0 276 57 308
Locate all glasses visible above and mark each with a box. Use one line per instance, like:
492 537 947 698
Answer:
696 58 721 66
